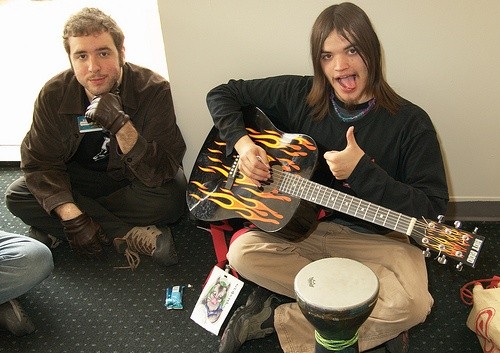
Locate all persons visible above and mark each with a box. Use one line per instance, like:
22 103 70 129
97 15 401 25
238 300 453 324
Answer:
206 2 449 353
6 8 188 267
0 230 54 338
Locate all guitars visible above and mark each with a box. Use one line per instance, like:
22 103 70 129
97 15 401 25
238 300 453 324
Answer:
187 105 486 271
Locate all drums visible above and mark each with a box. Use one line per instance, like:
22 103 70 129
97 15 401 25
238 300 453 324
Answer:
294 258 380 353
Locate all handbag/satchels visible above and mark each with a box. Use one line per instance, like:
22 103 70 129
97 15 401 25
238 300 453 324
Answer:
459 276 500 353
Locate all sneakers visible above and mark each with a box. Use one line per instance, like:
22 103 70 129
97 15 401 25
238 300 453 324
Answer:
1 300 34 337
29 227 62 249
218 289 285 353
388 334 409 353
113 225 178 266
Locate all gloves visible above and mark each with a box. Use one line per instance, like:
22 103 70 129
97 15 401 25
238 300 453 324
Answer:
60 211 112 263
84 94 129 137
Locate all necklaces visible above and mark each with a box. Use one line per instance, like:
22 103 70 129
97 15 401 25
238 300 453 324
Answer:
329 90 377 123
